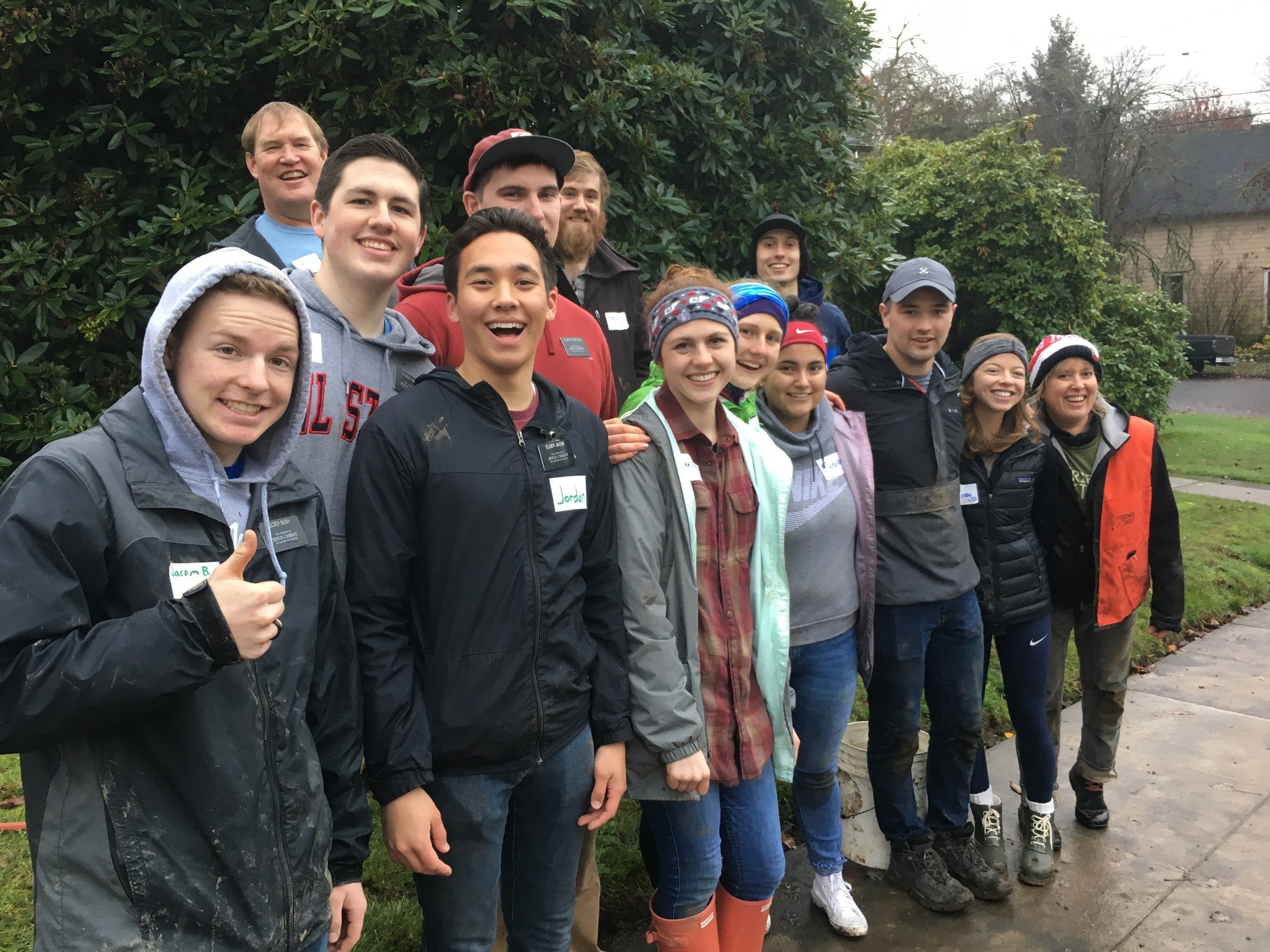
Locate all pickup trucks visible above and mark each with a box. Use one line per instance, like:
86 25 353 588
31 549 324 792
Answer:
1174 333 1239 377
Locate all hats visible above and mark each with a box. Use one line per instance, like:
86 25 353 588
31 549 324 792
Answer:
463 128 575 192
1028 334 1102 390
882 257 957 304
753 214 805 240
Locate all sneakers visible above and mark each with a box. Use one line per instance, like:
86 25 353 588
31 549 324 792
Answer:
887 828 974 914
1069 762 1109 828
811 871 868 936
932 822 1013 900
1018 782 1062 851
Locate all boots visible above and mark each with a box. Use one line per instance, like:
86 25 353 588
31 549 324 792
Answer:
970 793 1008 878
1019 797 1054 886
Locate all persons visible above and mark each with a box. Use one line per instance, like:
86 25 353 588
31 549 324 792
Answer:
1026 333 1185 829
343 204 635 952
826 258 1014 913
959 333 1058 887
1 246 375 952
214 97 876 952
